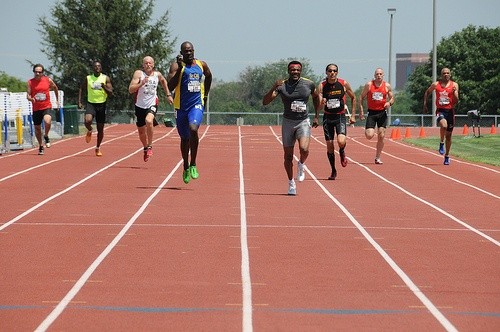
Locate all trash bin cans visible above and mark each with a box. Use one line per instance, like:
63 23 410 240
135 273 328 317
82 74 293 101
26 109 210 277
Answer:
53 105 79 135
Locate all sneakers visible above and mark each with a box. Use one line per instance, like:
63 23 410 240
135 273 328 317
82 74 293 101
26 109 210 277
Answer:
189 164 199 179
38 146 44 154
444 154 450 165
44 135 50 148
298 160 306 182
95 146 102 156
439 143 444 154
288 178 296 195
147 145 153 156
86 130 93 143
328 169 337 180
339 149 347 167
183 163 190 182
144 147 150 161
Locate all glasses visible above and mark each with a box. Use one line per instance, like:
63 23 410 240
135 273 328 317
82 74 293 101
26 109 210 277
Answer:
329 70 337 72
35 72 41 73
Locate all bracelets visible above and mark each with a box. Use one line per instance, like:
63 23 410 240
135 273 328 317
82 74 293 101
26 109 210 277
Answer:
388 101 391 106
204 96 208 98
166 93 171 97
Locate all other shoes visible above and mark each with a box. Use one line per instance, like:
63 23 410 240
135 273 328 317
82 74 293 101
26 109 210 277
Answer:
374 158 382 164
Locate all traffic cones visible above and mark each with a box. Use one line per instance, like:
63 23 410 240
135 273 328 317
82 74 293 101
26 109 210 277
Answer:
389 129 397 140
403 127 411 139
394 128 402 140
490 125 496 134
418 127 426 139
463 125 468 134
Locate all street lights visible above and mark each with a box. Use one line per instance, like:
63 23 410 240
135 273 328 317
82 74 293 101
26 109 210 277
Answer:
387 8 397 83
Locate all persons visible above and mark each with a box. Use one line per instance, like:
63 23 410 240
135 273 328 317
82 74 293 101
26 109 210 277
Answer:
317 64 356 180
129 56 174 162
423 68 459 165
27 64 59 155
263 61 320 195
78 61 112 156
167 42 212 184
359 67 394 165
